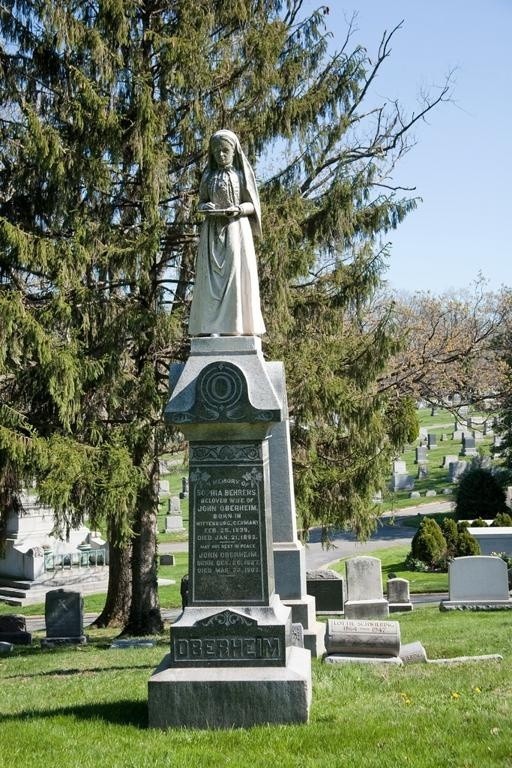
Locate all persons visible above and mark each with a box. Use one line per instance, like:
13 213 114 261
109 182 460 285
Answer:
181 574 188 611
188 129 267 338
